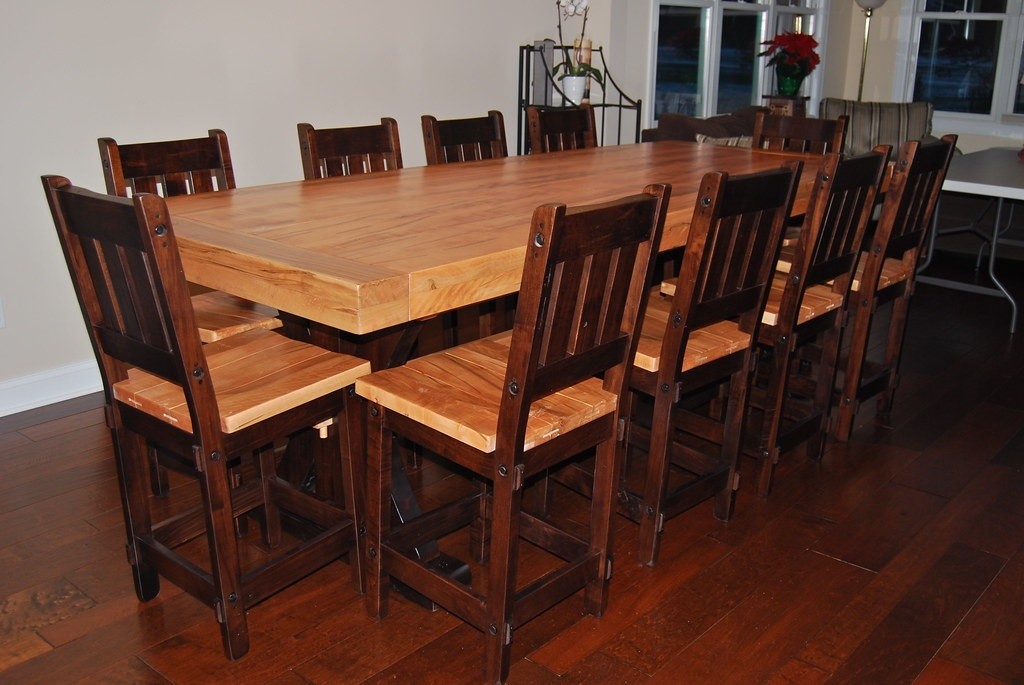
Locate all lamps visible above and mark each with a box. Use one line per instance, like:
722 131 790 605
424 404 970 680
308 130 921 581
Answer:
854 0 887 102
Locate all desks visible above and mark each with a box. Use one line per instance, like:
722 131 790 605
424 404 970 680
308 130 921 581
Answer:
161 139 897 611
913 146 1024 334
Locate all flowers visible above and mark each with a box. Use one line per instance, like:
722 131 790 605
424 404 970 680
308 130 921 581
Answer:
531 0 604 93
757 28 820 80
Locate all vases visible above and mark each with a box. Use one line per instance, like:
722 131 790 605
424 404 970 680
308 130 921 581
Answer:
777 69 804 95
562 77 586 106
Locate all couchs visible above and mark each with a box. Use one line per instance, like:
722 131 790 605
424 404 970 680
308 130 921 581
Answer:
641 105 774 150
820 97 963 222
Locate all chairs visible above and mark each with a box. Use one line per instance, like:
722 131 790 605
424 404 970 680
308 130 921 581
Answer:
533 159 805 569
747 135 958 442
355 183 674 685
98 103 599 539
41 174 369 663
661 144 895 501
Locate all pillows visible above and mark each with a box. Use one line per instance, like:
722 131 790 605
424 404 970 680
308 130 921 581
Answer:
658 106 771 150
